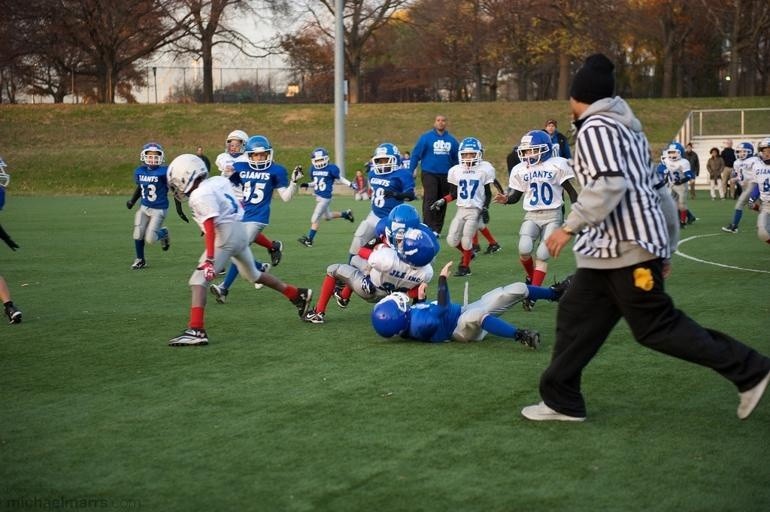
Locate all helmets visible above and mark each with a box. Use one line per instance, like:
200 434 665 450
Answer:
661 144 683 163
373 143 403 167
458 137 485 165
139 130 273 192
371 291 410 339
758 138 770 165
734 142 754 160
517 130 552 170
311 149 329 165
388 205 439 265
0 159 11 187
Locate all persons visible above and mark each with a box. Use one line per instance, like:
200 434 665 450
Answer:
1 156 24 326
166 131 313 346
297 114 580 352
125 142 190 271
655 138 770 241
520 54 770 423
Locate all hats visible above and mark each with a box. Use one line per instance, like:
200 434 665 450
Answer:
571 53 615 104
546 118 556 128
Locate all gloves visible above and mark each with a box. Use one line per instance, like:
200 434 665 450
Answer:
196 258 217 281
3 233 19 251
126 200 133 209
383 191 397 199
291 165 305 183
674 181 681 185
430 198 446 211
179 211 188 223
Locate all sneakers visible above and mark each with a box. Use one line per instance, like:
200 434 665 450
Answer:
525 275 572 308
737 369 770 418
160 227 169 251
131 258 145 269
456 243 501 276
210 281 231 304
342 209 354 222
521 400 587 421
295 286 350 324
686 216 696 225
515 328 541 351
7 307 22 325
268 240 283 266
168 328 209 346
254 263 272 290
297 236 313 248
722 224 739 234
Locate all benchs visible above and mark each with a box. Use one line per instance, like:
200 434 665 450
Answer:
673 138 770 191
